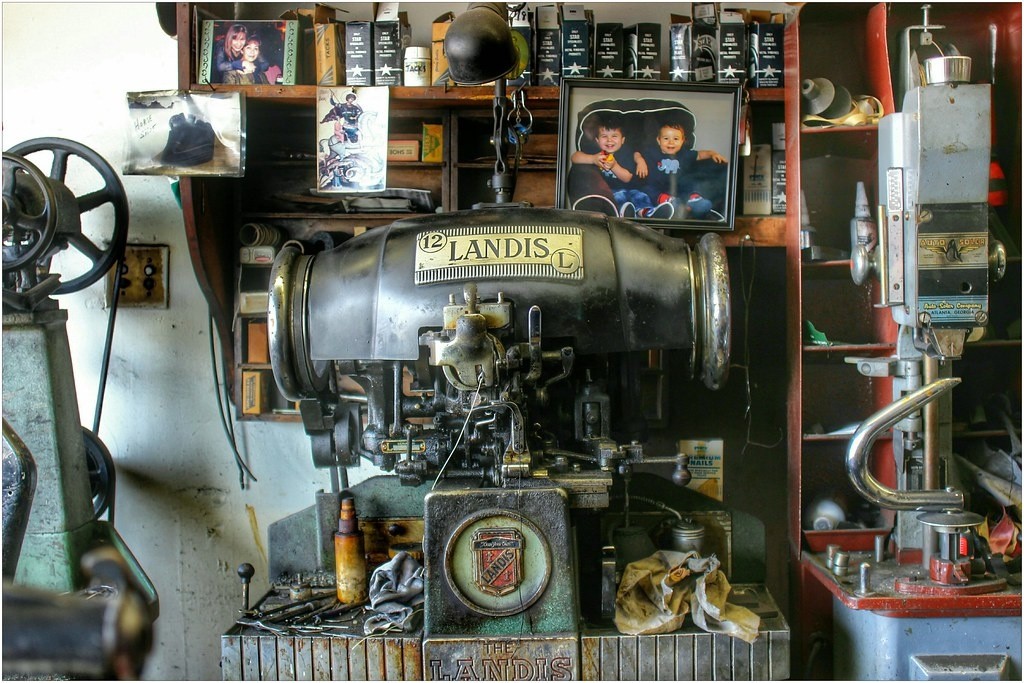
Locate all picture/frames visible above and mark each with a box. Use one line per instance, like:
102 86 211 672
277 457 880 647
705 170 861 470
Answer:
559 77 742 231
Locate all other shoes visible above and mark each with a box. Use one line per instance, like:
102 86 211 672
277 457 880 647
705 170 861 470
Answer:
646 203 674 219
669 197 681 216
687 196 712 217
618 202 635 218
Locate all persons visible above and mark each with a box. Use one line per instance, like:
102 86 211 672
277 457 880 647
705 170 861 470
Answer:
210 24 281 84
572 117 729 219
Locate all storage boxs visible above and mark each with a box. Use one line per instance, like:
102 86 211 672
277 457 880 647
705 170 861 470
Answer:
242 370 271 416
193 1 786 216
248 320 269 364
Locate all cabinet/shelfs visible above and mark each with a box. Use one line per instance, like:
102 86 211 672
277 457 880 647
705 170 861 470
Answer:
158 2 786 424
786 3 1022 680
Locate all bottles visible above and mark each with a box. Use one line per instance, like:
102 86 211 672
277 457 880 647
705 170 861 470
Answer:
403 47 431 86
850 181 877 254
801 190 816 249
804 482 850 531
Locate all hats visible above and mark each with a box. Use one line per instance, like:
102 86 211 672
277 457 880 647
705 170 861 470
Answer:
247 31 262 43
